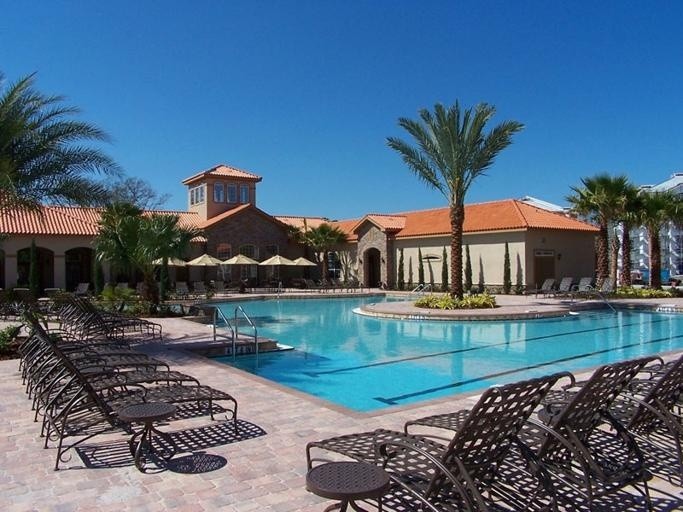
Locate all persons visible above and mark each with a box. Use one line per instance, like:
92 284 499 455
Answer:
238 272 250 294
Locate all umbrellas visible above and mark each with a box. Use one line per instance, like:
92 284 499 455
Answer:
256 254 296 283
148 254 186 291
215 252 259 286
288 256 318 280
184 251 224 292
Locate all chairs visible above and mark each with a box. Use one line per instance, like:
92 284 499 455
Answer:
304 277 370 294
0 280 234 298
523 276 616 299
